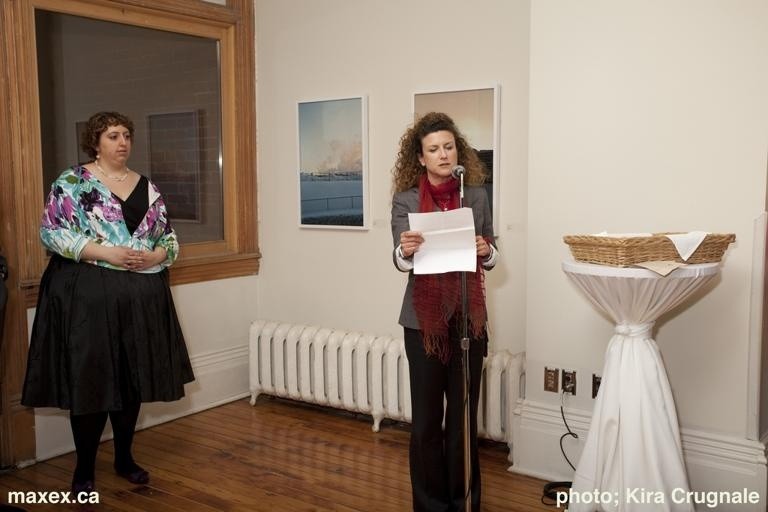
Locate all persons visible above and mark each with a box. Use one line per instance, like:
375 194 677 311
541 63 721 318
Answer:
392 113 498 512
20 113 196 501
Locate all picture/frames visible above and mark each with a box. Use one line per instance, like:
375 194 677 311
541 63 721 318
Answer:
410 82 501 243
294 92 371 231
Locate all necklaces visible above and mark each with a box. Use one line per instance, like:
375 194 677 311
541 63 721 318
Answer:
95 160 130 181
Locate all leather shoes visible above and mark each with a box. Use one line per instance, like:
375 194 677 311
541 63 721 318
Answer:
71 472 97 505
113 461 150 484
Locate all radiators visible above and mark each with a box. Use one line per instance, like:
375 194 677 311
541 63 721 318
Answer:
248 319 526 447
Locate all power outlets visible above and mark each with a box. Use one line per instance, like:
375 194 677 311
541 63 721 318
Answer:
592 374 603 399
562 370 576 396
544 366 558 393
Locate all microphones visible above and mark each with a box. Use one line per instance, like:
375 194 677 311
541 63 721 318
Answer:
450 165 464 176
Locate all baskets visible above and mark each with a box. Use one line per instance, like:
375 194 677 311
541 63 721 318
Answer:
562 232 736 268
